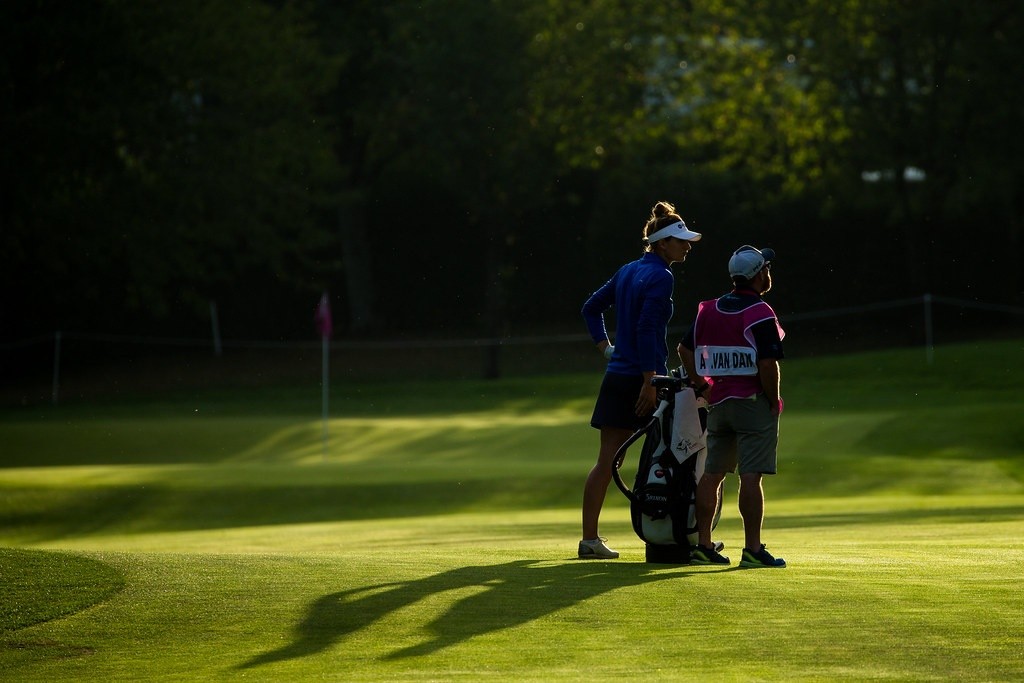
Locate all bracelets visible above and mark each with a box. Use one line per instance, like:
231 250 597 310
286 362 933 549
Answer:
698 381 710 393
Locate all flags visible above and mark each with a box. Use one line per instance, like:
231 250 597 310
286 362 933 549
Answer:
317 302 337 339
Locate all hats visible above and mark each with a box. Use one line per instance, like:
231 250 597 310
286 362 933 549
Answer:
728 245 776 284
649 221 702 244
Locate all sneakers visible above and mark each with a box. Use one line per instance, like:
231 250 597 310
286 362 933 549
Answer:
690 546 730 564
739 544 785 568
579 538 618 559
712 541 724 552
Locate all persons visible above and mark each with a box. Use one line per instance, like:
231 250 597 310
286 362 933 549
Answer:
578 201 724 559
678 244 786 569
